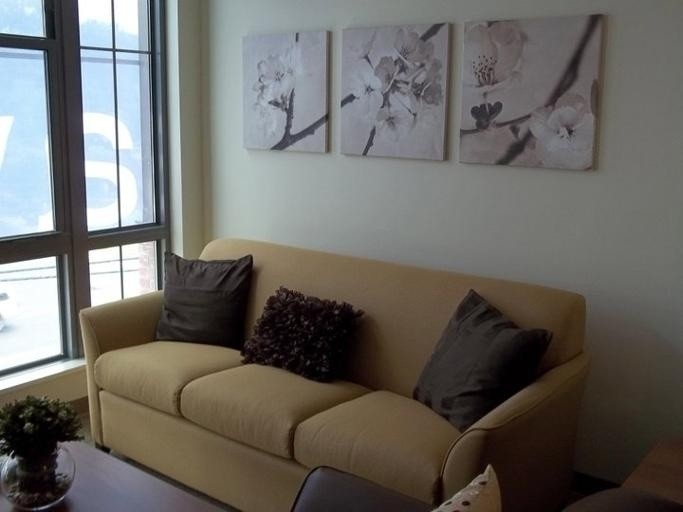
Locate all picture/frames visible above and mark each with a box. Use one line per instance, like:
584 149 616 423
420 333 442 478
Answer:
341 27 446 162
458 14 602 173
242 32 327 155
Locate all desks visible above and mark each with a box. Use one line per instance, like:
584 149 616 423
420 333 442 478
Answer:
622 439 681 509
2 440 223 511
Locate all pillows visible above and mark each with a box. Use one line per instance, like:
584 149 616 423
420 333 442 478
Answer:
155 252 255 351
240 285 367 384
409 288 553 433
430 463 503 512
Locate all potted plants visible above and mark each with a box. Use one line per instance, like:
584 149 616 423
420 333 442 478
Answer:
0 394 84 509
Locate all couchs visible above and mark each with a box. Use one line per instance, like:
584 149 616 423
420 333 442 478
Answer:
79 237 592 511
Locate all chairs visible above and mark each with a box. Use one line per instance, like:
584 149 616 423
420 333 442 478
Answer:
291 463 682 511
